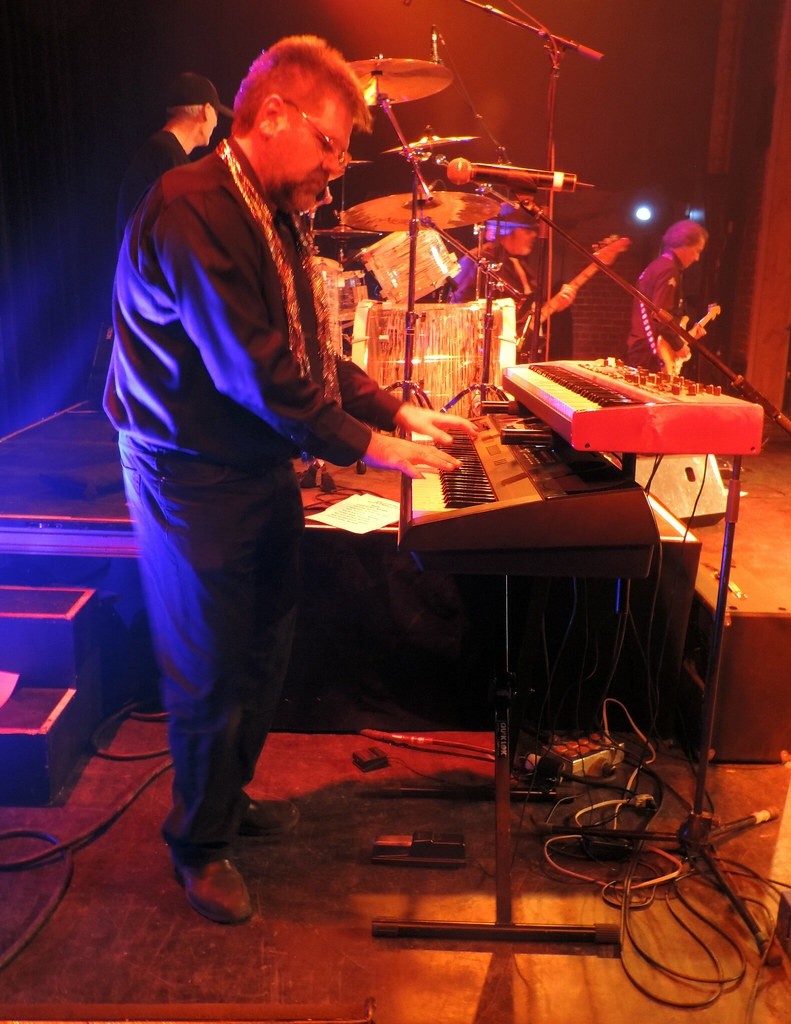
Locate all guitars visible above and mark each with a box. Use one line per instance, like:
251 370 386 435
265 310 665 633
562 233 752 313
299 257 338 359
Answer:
657 302 721 376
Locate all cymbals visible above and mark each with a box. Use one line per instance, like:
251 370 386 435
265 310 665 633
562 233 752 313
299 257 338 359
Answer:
339 191 502 231
308 224 383 239
380 125 482 158
346 53 453 106
346 160 374 169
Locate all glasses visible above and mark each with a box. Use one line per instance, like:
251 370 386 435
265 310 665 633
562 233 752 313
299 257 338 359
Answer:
280 96 352 168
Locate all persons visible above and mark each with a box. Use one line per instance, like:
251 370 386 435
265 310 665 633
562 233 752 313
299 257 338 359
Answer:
447 201 574 361
117 73 232 237
103 37 478 924
628 221 705 378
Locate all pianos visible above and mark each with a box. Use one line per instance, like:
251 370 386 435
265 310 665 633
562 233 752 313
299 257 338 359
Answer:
501 356 764 456
373 411 662 949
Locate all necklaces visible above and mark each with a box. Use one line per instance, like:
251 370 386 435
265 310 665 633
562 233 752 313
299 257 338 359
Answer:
216 138 342 469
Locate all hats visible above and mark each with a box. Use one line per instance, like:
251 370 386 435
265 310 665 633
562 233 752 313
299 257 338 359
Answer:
165 71 233 118
487 203 536 228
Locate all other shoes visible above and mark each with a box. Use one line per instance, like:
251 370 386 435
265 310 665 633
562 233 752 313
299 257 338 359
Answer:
171 851 252 924
235 797 299 834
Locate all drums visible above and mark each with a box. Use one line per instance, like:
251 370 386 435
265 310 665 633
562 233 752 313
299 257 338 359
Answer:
354 232 462 305
351 297 517 418
314 257 344 271
320 269 369 322
